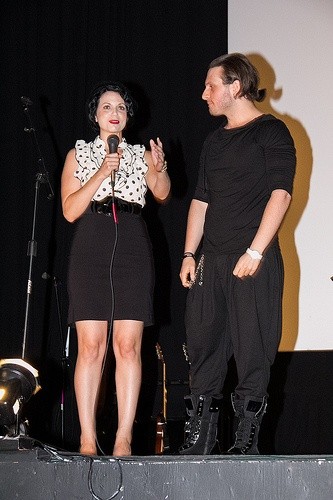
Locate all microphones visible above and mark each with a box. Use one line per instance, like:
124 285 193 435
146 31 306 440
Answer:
107 134 120 185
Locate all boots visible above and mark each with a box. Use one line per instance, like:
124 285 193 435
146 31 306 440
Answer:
176 394 223 454
226 393 266 456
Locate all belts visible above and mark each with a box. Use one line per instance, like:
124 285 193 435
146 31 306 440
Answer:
87 198 143 218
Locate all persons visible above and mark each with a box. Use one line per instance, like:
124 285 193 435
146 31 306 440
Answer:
60 83 170 457
179 51 297 455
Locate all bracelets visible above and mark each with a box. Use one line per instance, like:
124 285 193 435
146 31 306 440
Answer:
157 161 168 173
182 252 194 258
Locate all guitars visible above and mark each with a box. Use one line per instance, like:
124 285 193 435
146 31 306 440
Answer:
155 341 177 455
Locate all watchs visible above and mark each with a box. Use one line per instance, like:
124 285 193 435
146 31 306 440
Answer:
246 248 263 261
